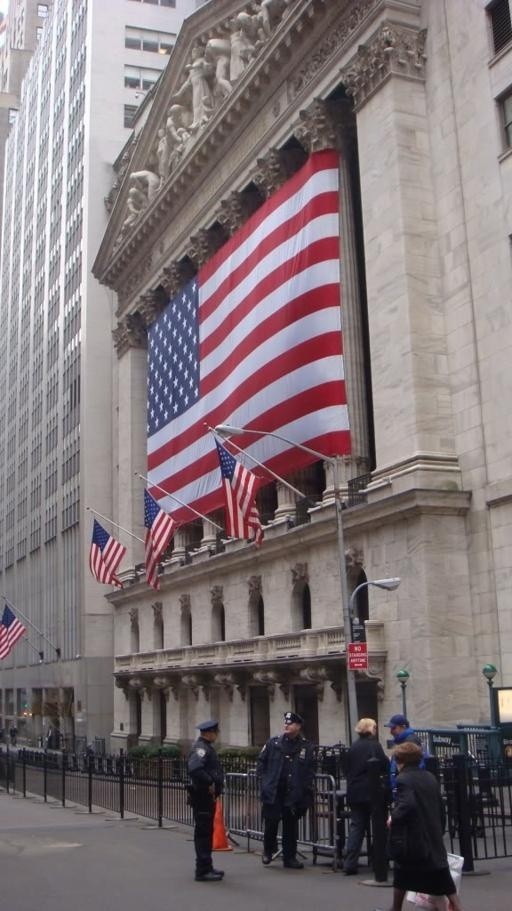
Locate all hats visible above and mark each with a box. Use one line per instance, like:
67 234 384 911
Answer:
195 720 221 732
384 715 407 727
284 711 304 724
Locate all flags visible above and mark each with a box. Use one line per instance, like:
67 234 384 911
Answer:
88 517 128 592
144 147 355 536
143 486 182 594
211 431 266 549
0 601 28 664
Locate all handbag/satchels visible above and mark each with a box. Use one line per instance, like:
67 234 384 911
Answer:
388 820 414 864
349 769 385 808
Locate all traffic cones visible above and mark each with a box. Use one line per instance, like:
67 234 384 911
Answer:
211 800 233 851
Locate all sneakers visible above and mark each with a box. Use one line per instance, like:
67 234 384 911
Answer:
262 853 272 863
342 866 358 874
283 856 303 868
196 870 224 880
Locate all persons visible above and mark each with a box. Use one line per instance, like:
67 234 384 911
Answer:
360 714 428 890
340 718 390 875
255 712 315 870
9 724 20 748
186 720 226 882
374 741 464 911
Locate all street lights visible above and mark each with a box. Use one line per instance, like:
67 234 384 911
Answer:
215 424 404 745
